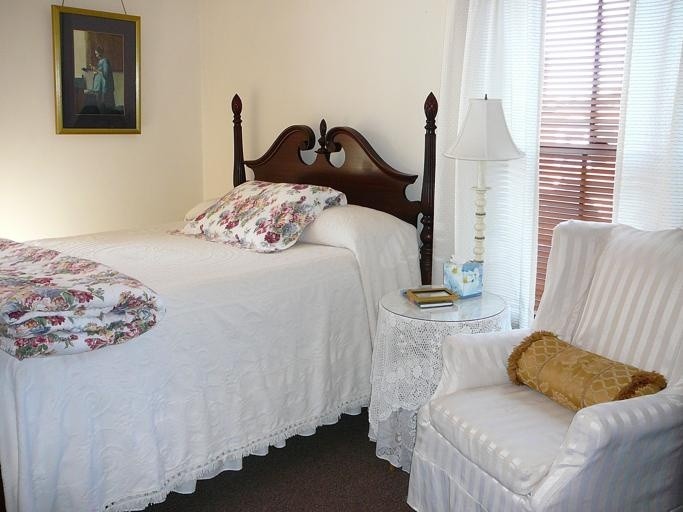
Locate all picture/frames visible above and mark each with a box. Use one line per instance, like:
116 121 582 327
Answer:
50 4 142 135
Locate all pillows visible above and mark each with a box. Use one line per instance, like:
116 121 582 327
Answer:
179 179 349 253
505 329 668 414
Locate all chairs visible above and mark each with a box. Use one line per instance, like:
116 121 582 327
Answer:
403 216 682 512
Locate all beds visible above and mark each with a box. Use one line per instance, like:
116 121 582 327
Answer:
0 90 439 512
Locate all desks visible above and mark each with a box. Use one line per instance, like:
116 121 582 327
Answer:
368 284 512 477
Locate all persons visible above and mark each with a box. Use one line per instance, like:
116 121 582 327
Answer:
91 47 115 114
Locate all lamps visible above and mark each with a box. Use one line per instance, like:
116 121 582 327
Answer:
441 91 528 297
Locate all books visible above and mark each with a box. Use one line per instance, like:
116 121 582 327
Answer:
403 292 453 309
